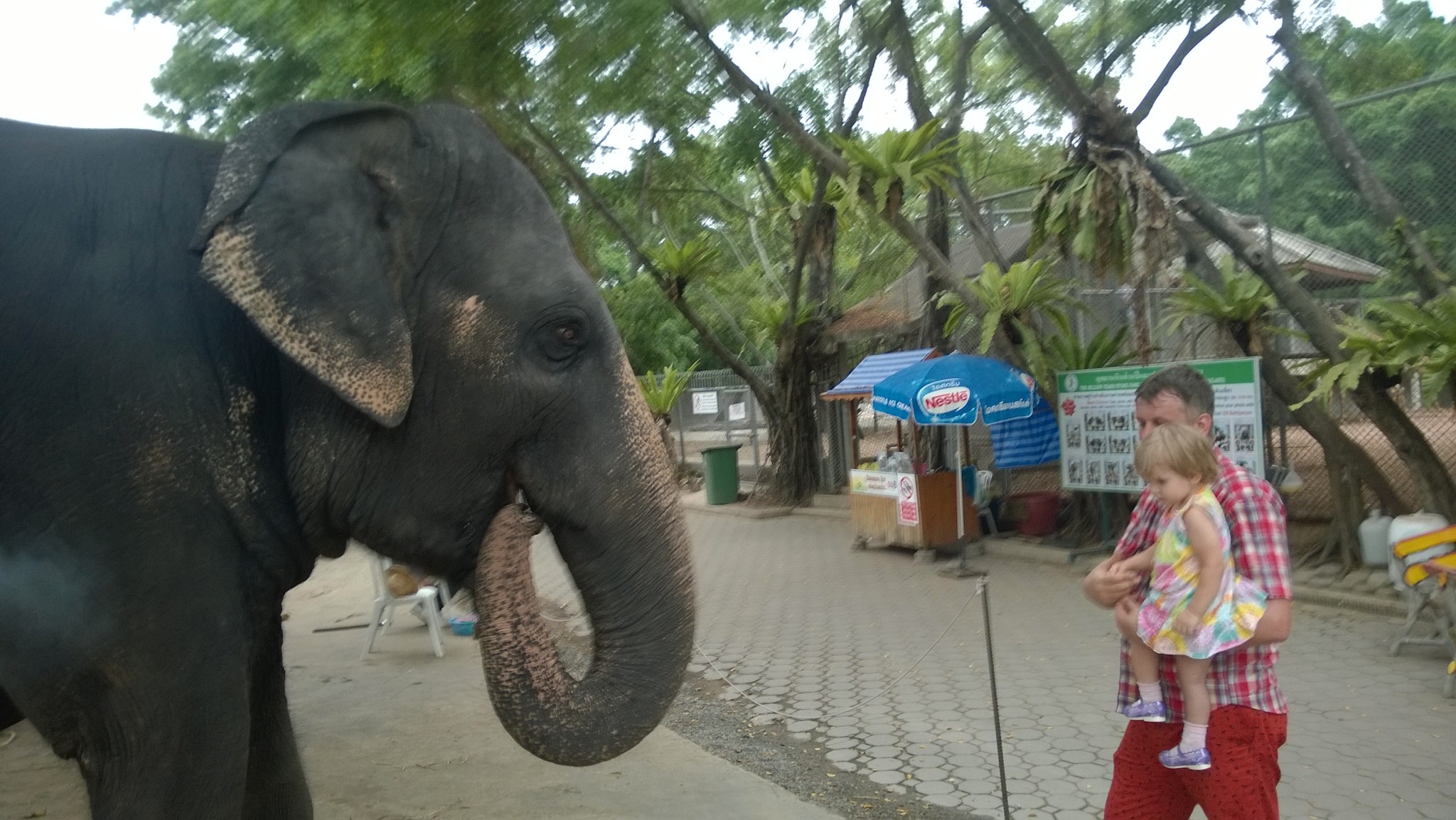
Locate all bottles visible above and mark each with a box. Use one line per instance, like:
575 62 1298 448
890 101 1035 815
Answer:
1358 509 1392 567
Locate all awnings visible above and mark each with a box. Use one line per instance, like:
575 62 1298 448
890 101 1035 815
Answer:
820 345 949 402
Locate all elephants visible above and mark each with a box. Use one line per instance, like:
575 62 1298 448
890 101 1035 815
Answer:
0 101 696 819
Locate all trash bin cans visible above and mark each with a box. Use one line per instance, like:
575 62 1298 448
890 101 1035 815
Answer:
701 442 743 505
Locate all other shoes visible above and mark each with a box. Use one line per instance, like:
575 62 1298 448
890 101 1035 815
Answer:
408 604 449 628
1159 748 1212 771
1121 698 1166 723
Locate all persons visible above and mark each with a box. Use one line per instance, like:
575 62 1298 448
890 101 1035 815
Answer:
1108 421 1268 770
1083 364 1292 820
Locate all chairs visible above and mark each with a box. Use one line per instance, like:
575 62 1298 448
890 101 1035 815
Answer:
972 469 998 537
362 548 444 657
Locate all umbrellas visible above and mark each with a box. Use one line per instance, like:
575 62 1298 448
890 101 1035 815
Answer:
873 350 1038 580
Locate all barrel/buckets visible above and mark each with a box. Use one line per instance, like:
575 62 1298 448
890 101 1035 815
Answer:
1388 511 1455 590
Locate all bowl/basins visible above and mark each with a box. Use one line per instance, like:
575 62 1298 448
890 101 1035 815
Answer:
449 617 474 637
